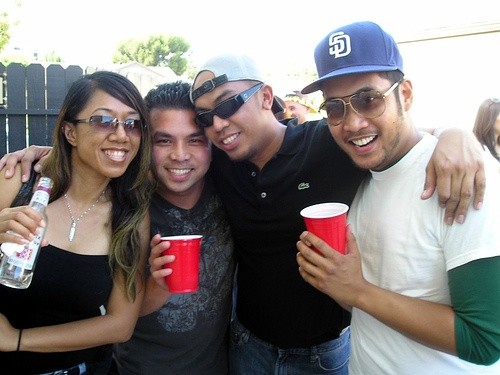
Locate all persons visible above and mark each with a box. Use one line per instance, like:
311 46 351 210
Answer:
109 81 235 375
296 20 500 375
280 87 319 125
0 52 487 375
0 71 157 375
472 97 500 164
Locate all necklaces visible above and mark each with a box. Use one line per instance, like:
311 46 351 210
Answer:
63 191 104 241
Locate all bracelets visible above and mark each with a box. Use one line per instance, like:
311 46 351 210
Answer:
17 328 23 353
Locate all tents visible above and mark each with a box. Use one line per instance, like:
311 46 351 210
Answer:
104 61 185 99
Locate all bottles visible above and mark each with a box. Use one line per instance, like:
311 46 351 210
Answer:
0 177 54 289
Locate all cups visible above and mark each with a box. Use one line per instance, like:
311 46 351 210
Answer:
300 203 349 255
160 235 203 294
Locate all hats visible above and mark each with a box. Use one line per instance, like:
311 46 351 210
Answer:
189 46 287 115
284 90 318 114
301 21 405 95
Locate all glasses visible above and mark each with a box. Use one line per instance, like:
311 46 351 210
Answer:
318 76 404 126
193 82 266 130
487 96 500 109
69 115 146 137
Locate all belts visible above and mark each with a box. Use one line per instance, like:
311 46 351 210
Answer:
60 363 92 375
